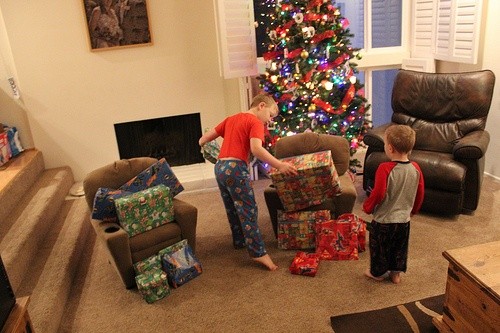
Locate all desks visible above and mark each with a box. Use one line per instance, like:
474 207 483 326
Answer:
432 239 500 333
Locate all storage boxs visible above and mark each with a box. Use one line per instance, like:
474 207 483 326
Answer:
268 150 343 211
290 251 320 277
278 209 331 250
133 239 202 305
91 187 135 222
115 158 184 200
115 183 176 238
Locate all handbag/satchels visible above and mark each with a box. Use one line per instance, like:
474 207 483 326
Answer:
316 220 366 260
337 213 367 253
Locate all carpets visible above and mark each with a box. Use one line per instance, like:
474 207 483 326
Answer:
329 293 445 333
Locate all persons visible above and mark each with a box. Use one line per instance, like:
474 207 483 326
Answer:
199 93 298 271
360 125 425 283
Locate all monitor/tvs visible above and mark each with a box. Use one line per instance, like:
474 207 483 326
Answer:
114 112 205 166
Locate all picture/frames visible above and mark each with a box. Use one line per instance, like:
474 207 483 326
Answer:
81 0 154 52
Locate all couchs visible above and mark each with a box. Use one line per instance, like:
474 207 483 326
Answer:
82 157 197 292
362 68 496 216
264 132 356 237
